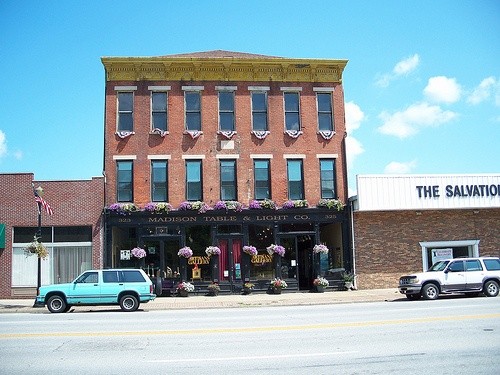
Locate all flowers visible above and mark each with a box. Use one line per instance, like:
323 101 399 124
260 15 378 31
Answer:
176 280 194 293
205 245 221 256
145 202 173 214
248 199 280 211
108 202 137 215
312 275 329 286
131 247 147 259
178 200 209 215
214 200 244 212
207 282 219 290
313 244 328 256
319 199 347 211
282 200 310 210
177 246 194 260
243 246 257 256
270 278 287 288
266 243 286 258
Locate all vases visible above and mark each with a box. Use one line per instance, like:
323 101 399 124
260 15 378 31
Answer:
210 288 218 296
315 286 325 293
178 290 188 297
273 288 281 294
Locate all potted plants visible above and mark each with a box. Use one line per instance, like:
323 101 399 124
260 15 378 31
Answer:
243 282 254 294
21 241 49 261
341 269 358 291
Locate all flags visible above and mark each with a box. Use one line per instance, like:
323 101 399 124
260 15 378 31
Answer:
33 188 54 216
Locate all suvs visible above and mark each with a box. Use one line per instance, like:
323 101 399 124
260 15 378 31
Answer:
398 257 500 301
37 268 156 313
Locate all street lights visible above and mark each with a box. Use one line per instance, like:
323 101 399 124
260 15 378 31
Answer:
30 182 47 309
